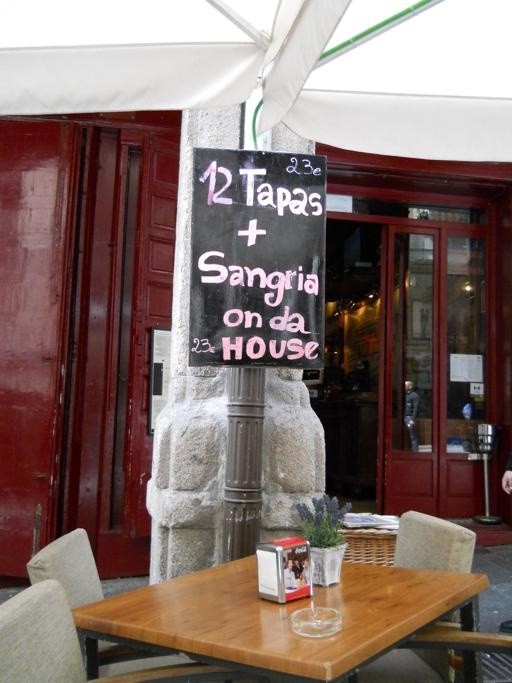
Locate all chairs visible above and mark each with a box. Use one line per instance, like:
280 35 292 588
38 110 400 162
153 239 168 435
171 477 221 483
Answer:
334 508 478 682
25 526 200 682
1 577 272 682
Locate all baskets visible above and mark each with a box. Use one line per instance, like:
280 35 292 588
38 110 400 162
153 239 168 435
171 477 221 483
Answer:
332 510 403 566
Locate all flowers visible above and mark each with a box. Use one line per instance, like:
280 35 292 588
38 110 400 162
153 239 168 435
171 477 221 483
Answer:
294 489 354 552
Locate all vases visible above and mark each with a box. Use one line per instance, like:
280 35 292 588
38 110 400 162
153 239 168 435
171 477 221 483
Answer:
307 540 349 588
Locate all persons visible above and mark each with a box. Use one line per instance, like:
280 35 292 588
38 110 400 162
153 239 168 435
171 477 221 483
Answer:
281 554 311 593
498 442 512 637
460 401 473 451
404 378 422 452
